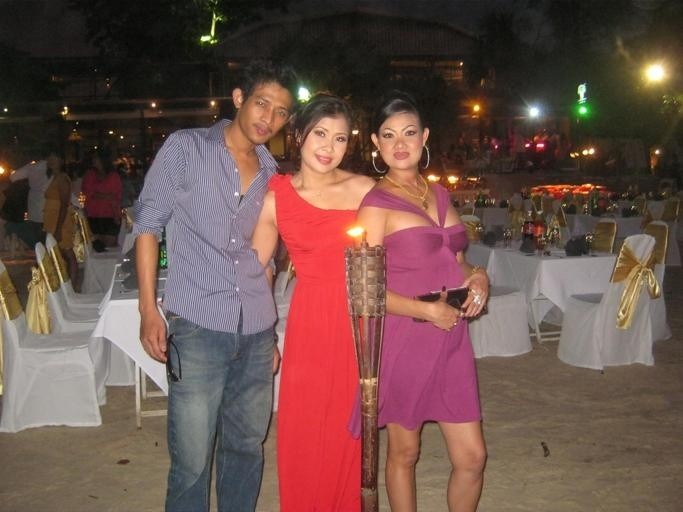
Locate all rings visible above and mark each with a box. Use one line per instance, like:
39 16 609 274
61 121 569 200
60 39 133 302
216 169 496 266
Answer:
472 295 481 306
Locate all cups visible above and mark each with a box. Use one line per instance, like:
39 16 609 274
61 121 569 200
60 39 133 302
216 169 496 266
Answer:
503 227 513 250
474 222 485 243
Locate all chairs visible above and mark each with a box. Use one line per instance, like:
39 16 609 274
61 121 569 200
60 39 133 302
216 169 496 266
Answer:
1 263 109 434
72 209 133 295
450 192 682 368
23 232 140 386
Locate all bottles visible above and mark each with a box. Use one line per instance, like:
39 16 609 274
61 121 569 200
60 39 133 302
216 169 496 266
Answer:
582 201 592 214
533 206 545 254
157 226 167 268
474 190 484 208
559 201 568 213
520 210 535 251
590 184 598 209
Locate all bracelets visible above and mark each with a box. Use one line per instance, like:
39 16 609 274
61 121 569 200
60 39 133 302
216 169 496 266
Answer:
470 262 487 276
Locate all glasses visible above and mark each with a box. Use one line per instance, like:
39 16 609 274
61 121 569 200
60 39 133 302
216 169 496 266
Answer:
167 335 181 382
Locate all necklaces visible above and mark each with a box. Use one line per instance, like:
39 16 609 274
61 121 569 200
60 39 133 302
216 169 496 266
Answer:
384 172 429 211
297 168 338 201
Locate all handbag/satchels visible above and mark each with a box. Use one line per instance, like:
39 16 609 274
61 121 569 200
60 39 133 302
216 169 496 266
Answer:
413 288 469 323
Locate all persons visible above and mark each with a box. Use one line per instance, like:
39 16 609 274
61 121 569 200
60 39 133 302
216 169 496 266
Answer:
0 125 146 293
432 121 568 174
132 55 298 511
249 92 380 512
346 91 492 512
